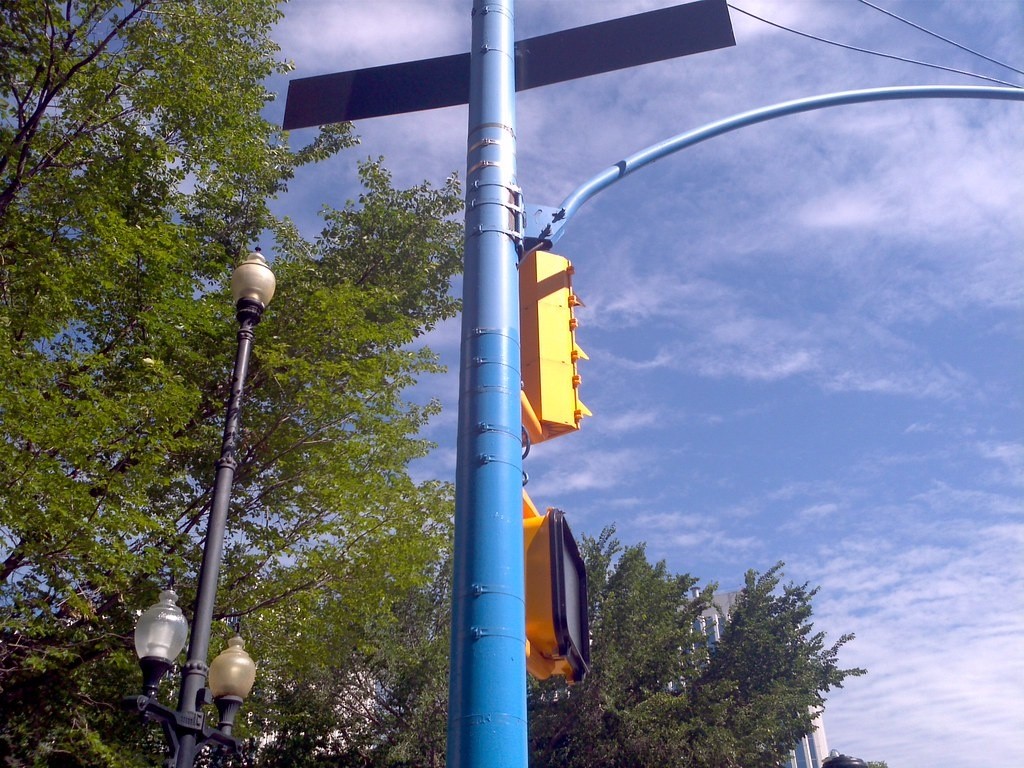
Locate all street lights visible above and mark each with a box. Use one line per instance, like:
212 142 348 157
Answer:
133 244 276 768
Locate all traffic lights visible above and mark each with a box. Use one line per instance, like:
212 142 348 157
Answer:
519 250 592 445
522 507 591 686
